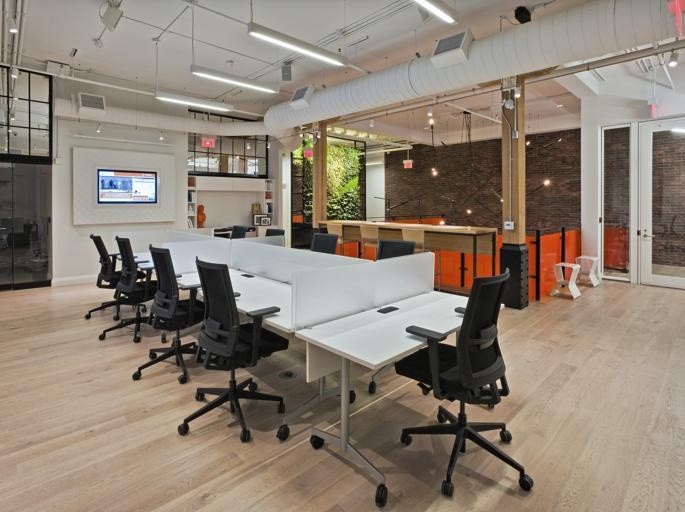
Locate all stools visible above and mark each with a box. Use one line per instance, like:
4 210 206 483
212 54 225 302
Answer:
575 254 602 290
548 261 582 301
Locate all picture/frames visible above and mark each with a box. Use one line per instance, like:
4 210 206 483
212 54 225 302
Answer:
252 214 268 226
259 217 271 226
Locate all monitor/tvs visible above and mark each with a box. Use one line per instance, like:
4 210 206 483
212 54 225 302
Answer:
97 169 157 204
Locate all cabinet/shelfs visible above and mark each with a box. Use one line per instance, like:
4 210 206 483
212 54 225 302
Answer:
255 178 275 216
187 174 199 229
314 221 502 290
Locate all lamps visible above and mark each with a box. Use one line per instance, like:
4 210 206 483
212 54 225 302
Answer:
368 119 375 128
158 130 166 141
190 62 281 94
98 0 124 33
153 89 235 114
316 131 322 139
266 142 272 149
247 20 350 70
304 133 310 139
668 48 679 67
244 139 255 153
0 1 23 134
95 120 106 134
414 2 460 26
426 111 435 124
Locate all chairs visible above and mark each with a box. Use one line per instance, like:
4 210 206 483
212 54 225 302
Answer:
84 220 542 512
